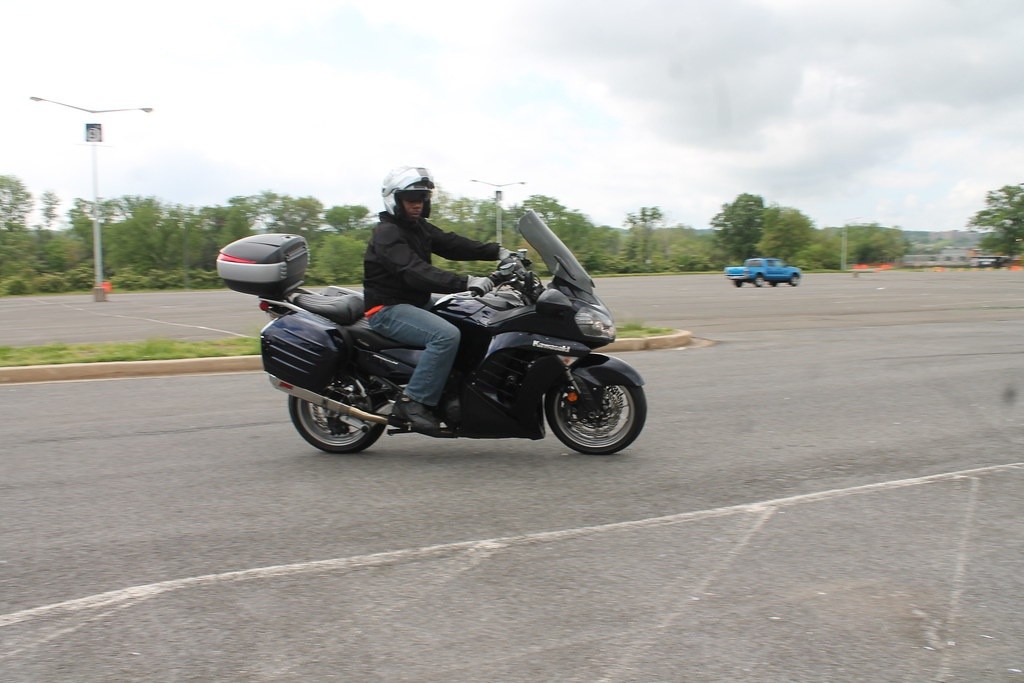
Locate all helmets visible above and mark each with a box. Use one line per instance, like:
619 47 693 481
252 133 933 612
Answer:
382 165 436 222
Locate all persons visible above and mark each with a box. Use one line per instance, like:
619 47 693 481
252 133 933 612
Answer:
364 166 518 437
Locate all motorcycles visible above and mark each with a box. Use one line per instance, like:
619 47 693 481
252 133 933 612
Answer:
216 209 648 455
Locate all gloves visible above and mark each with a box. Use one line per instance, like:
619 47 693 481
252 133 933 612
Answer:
498 247 518 263
467 275 494 297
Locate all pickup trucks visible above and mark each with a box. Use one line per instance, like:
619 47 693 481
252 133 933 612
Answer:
724 257 801 287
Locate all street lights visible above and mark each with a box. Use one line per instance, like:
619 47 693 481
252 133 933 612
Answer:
472 179 527 246
31 96 152 301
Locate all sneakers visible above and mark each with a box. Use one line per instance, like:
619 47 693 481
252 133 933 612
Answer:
392 395 438 433
